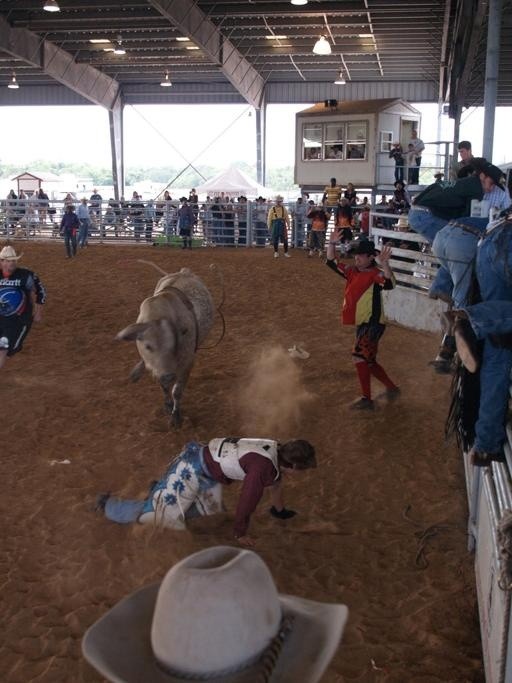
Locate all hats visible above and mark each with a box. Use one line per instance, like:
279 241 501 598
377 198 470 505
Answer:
81 545 349 683
346 238 379 255
66 189 99 210
180 197 190 202
237 195 284 202
470 161 506 191
394 180 405 188
0 246 25 260
433 171 444 178
395 219 411 227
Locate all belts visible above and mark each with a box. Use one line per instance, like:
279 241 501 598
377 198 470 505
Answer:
449 221 480 237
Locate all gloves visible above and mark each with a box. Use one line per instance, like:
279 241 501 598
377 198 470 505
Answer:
270 505 298 520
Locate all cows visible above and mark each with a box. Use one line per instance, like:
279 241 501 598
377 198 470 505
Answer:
113 258 214 431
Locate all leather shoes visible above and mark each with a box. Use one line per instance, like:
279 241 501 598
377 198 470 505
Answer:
437 307 483 374
468 443 507 466
428 290 453 303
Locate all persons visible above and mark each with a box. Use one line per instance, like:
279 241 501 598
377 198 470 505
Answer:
1 188 50 235
409 163 504 304
433 172 449 189
449 141 485 177
325 141 342 159
0 242 45 379
323 227 403 415
433 216 492 378
103 188 199 250
389 141 404 182
199 192 292 258
347 141 365 159
406 130 424 185
433 171 446 185
291 177 423 287
304 142 322 159
457 164 508 211
440 214 512 467
95 436 317 549
51 188 103 258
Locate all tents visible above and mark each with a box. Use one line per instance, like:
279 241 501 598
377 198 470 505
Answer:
199 166 263 200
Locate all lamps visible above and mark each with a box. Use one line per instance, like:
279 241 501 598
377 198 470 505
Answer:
42 0 61 13
313 36 331 55
115 36 127 54
7 71 19 89
333 71 347 86
160 69 172 86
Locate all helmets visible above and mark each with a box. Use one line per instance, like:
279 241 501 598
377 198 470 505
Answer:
279 438 316 469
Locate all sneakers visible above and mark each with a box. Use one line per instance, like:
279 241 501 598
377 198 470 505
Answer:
349 396 374 410
274 250 323 257
379 388 401 401
